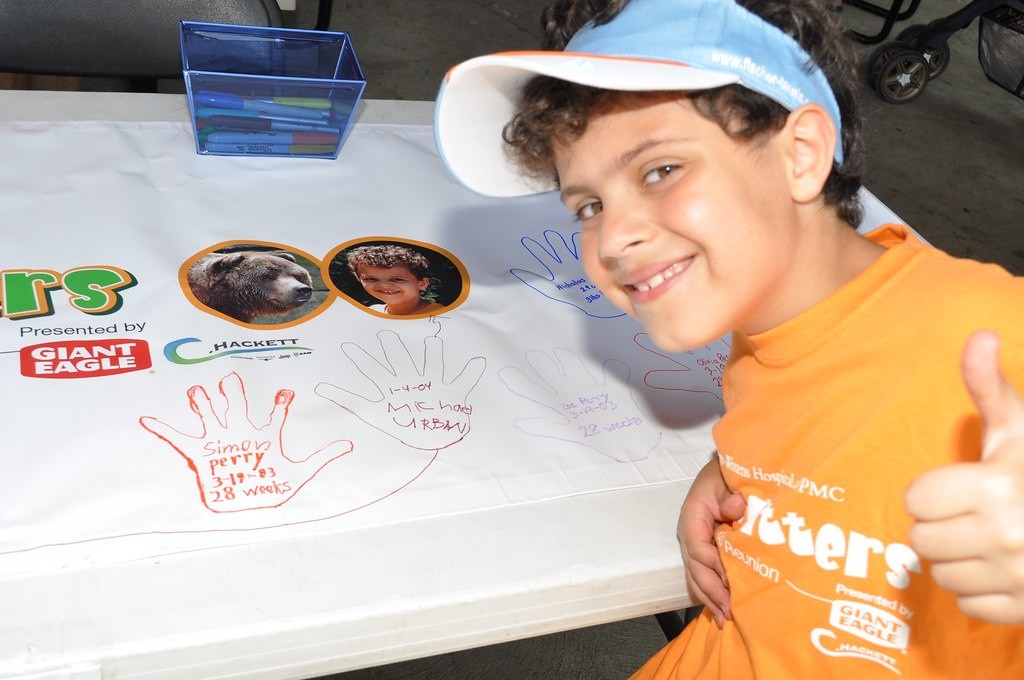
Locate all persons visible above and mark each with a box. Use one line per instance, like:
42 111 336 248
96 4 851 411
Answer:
434 0 1024 679
345 244 445 315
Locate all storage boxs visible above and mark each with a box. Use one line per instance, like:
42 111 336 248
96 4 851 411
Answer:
177 19 366 159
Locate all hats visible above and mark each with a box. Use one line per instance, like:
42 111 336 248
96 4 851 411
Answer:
432 0 844 199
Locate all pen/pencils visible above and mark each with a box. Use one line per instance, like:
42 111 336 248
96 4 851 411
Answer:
194 90 340 154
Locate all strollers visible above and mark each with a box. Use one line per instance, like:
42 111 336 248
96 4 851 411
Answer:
869 1 1024 104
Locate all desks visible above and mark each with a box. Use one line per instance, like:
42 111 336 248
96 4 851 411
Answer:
0 88 938 680
